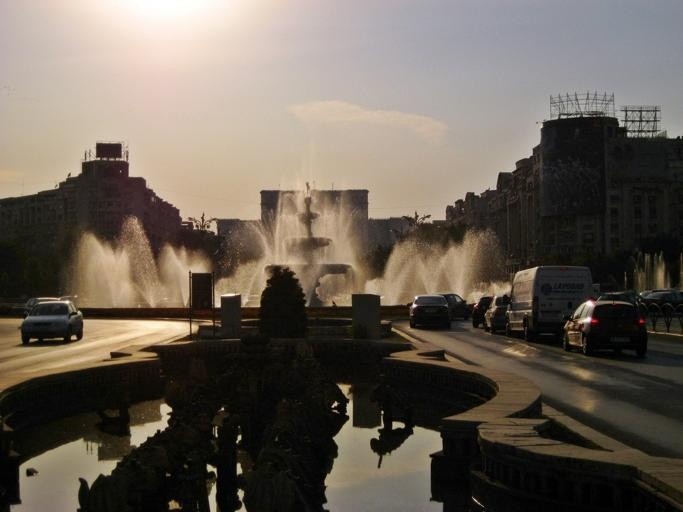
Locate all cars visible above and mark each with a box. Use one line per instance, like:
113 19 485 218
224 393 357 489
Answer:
408 296 449 330
442 294 471 320
471 265 682 358
23 296 60 320
21 300 82 343
